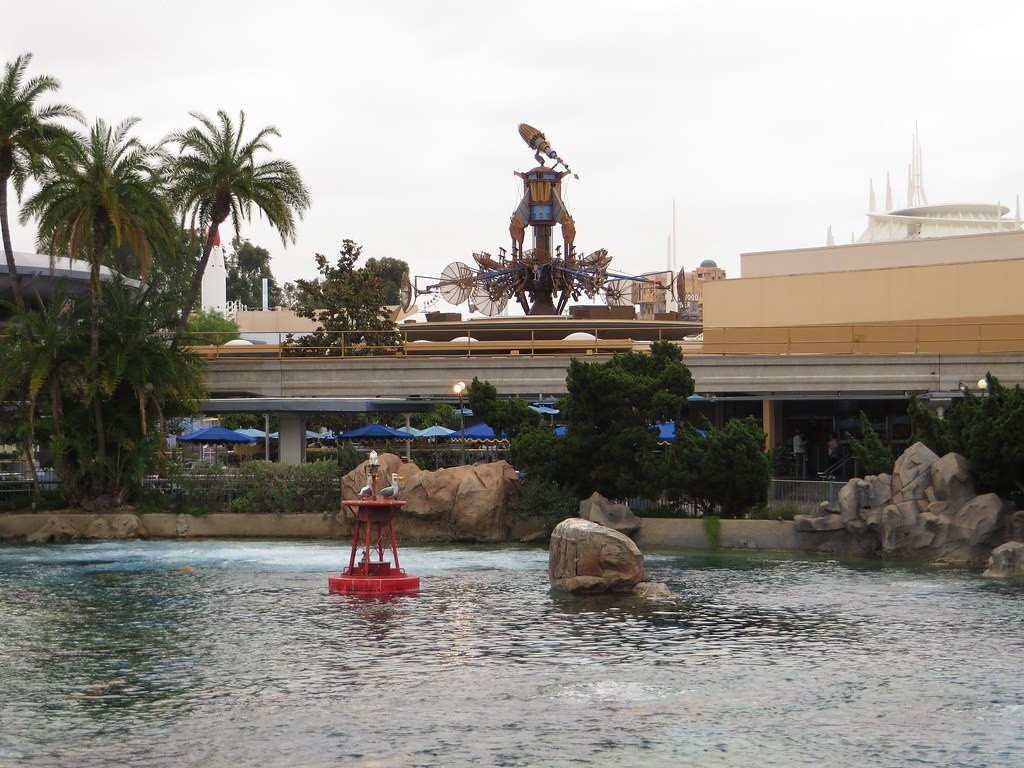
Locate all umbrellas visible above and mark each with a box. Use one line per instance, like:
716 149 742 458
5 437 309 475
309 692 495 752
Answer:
229 425 246 463
415 423 457 470
271 430 340 440
239 426 271 461
447 422 513 464
393 426 423 437
176 426 256 466
337 424 414 455
646 419 708 461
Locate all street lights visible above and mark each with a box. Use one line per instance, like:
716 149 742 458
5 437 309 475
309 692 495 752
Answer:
453 382 467 465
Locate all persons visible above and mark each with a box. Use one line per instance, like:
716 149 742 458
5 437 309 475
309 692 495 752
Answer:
826 433 839 481
794 432 810 477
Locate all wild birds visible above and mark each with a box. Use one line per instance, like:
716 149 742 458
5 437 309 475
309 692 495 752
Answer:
357 473 404 501
